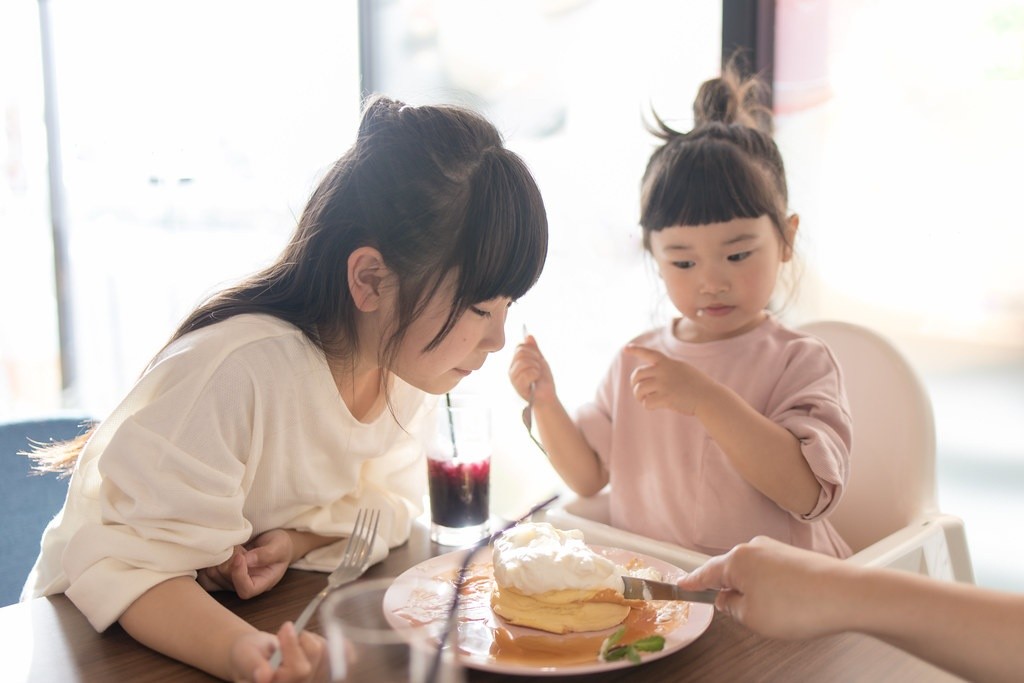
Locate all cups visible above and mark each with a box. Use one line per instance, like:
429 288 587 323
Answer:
323 578 464 683
423 397 490 544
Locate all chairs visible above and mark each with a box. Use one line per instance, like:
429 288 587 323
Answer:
546 318 974 585
0 417 102 609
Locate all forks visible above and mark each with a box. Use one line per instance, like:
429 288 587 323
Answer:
267 509 382 664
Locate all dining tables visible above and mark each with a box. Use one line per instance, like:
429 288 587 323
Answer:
0 525 969 683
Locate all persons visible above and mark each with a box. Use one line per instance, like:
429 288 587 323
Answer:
676 534 1024 683
20 95 549 683
508 79 852 559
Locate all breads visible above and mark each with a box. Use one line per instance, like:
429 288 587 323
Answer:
489 522 646 631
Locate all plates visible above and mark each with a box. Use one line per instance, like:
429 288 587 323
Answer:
381 540 715 676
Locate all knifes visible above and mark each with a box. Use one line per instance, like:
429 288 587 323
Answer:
621 577 720 604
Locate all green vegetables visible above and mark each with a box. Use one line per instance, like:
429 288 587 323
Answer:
603 628 664 662
